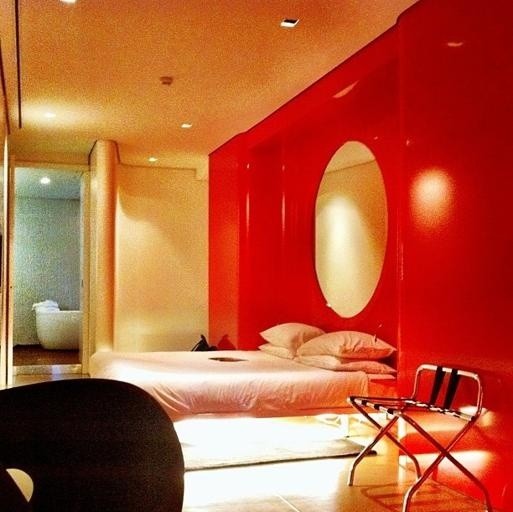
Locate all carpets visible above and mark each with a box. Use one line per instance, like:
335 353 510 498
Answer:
173 417 377 472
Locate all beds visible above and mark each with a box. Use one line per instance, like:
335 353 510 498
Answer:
87 350 397 421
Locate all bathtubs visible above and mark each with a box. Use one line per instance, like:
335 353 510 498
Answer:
35 311 82 349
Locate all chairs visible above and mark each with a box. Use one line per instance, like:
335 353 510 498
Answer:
0 378 184 512
347 364 494 512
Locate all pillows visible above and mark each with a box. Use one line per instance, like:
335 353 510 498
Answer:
298 330 396 359
259 322 325 349
257 343 298 359
295 355 397 373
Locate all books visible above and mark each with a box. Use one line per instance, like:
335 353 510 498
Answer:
207 357 247 362
